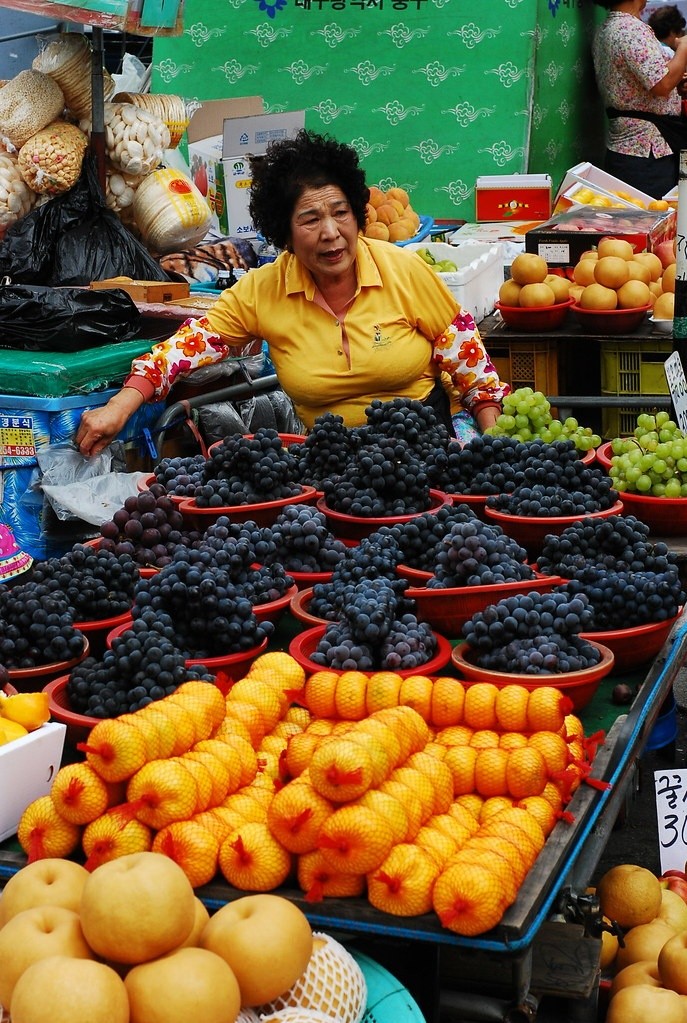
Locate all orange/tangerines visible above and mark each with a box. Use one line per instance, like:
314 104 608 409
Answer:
0 652 584 936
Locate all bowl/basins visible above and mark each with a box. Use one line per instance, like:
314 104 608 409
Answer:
495 295 576 330
569 291 656 330
3 433 687 748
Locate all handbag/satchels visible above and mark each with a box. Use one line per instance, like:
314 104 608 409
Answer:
605 106 687 154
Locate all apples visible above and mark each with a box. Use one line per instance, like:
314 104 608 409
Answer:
415 249 463 271
658 876 687 903
663 870 685 881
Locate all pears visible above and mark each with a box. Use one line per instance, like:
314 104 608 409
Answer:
499 189 678 321
0 853 687 1023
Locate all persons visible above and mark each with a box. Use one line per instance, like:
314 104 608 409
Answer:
593 0 687 202
77 130 511 458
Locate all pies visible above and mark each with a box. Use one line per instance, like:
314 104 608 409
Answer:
0 32 213 250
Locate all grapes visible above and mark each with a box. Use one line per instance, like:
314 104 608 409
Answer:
0 387 687 721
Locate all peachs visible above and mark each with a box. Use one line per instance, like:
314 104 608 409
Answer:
364 186 419 242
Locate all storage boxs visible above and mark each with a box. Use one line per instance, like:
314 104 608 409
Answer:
187 96 304 242
0 336 163 561
0 716 69 841
394 162 681 326
483 340 561 421
601 339 677 440
